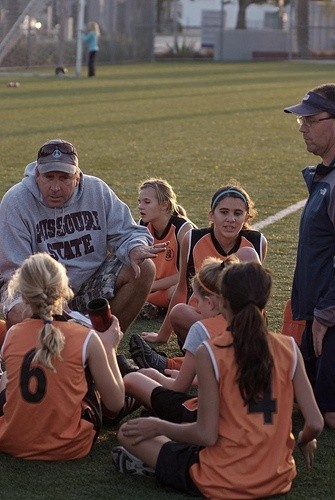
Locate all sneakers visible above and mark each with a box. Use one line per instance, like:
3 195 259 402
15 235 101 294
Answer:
117 354 139 378
110 446 157 479
137 302 168 321
129 333 169 374
108 396 152 429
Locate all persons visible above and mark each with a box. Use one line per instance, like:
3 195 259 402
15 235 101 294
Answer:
80 20 101 77
281 82 335 431
0 139 325 500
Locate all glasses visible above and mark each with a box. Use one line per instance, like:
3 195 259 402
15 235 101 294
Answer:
38 144 77 160
299 116 335 126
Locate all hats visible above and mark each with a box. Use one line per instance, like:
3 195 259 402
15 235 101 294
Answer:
36 139 79 174
284 92 335 117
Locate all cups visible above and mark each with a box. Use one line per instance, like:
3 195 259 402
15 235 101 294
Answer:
87 298 111 332
